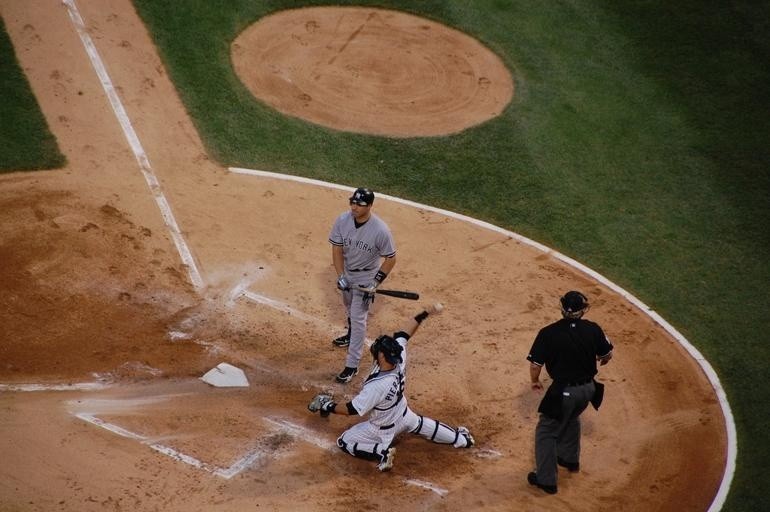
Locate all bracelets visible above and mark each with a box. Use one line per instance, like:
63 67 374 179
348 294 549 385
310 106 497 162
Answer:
374 270 387 283
415 311 429 324
327 402 337 414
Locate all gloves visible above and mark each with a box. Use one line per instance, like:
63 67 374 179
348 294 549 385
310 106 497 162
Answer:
337 276 352 292
357 280 379 304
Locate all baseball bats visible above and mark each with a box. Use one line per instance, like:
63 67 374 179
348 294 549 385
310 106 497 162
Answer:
349 286 419 300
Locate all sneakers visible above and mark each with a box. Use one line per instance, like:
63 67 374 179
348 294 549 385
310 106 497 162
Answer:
335 365 358 385
558 458 580 473
528 470 557 495
378 446 397 472
333 333 351 348
456 424 474 447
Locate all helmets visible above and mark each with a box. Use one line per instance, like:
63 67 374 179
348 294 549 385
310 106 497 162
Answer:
371 335 402 364
558 290 588 313
349 187 375 207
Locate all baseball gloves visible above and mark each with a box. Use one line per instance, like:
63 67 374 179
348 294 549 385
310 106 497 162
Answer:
307 393 337 417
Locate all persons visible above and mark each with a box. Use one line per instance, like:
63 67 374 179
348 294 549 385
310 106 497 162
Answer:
526 291 614 494
308 308 476 472
328 187 396 384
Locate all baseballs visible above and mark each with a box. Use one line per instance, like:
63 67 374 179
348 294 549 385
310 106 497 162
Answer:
435 303 444 313
